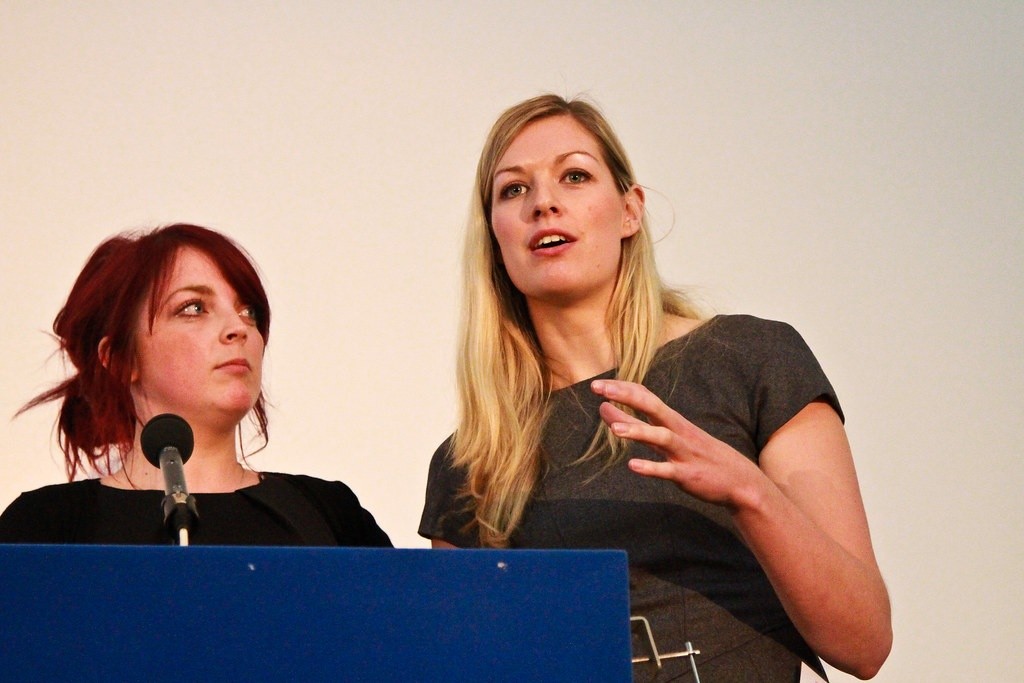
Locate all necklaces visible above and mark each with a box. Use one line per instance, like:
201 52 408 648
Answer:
134 462 244 493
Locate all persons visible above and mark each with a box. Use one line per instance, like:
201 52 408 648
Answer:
416 97 894 681
1 224 394 549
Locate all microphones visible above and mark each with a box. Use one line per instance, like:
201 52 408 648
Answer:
140 413 202 542
